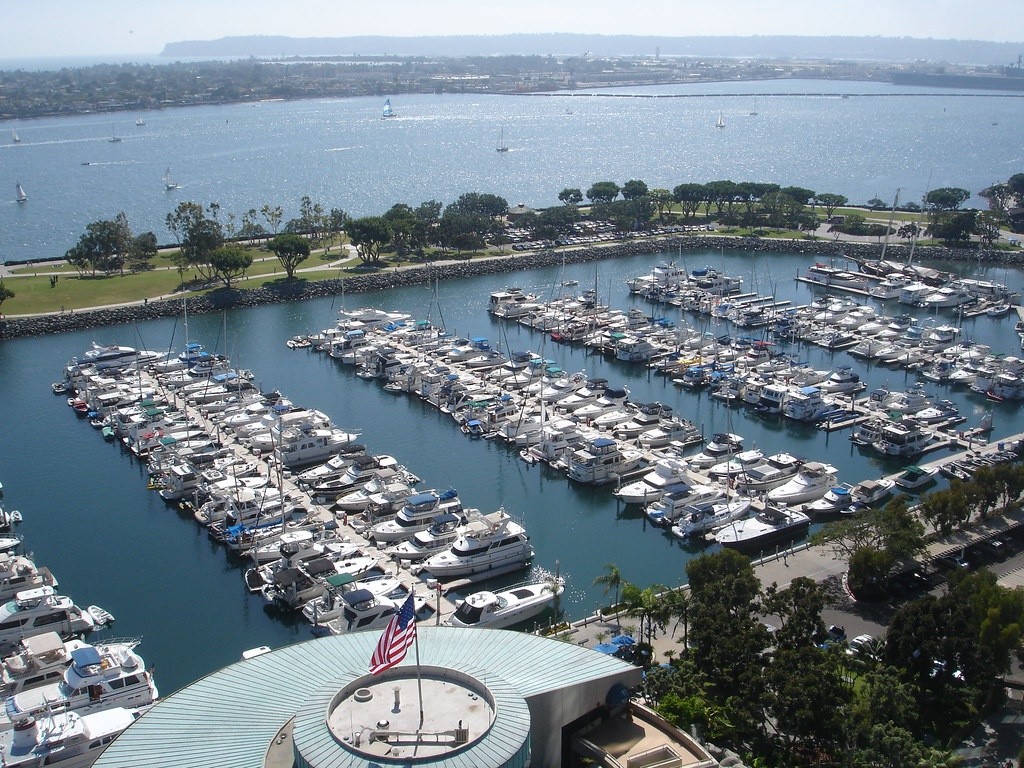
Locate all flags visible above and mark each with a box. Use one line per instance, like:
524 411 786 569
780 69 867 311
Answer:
367 592 417 676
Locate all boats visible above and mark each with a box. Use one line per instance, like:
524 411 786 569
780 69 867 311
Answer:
0 176 1024 767
382 98 397 118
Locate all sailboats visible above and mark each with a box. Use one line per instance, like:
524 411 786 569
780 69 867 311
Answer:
716 110 725 128
495 127 511 152
12 131 21 143
14 181 29 202
164 167 178 189
136 115 146 126
